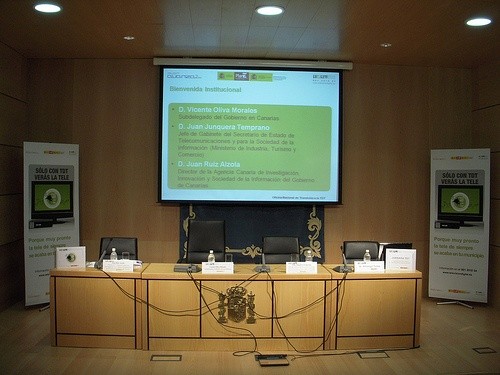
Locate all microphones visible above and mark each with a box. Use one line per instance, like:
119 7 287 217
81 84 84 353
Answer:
255 241 270 272
94 238 112 269
333 246 354 273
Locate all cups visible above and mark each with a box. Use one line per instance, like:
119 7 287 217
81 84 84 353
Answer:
122 252 129 260
225 254 232 262
291 254 300 262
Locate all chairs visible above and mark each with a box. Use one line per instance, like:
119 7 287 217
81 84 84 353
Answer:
343 241 379 263
186 219 226 264
261 236 300 264
99 236 138 260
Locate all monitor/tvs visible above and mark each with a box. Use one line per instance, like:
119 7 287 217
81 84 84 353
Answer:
31 181 74 224
438 184 483 227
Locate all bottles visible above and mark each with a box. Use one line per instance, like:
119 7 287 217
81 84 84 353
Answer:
363 249 371 261
306 251 312 262
208 250 215 262
111 248 117 260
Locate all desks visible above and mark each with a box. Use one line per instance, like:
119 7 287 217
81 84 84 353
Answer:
48 263 150 350
141 262 332 351
322 263 422 350
